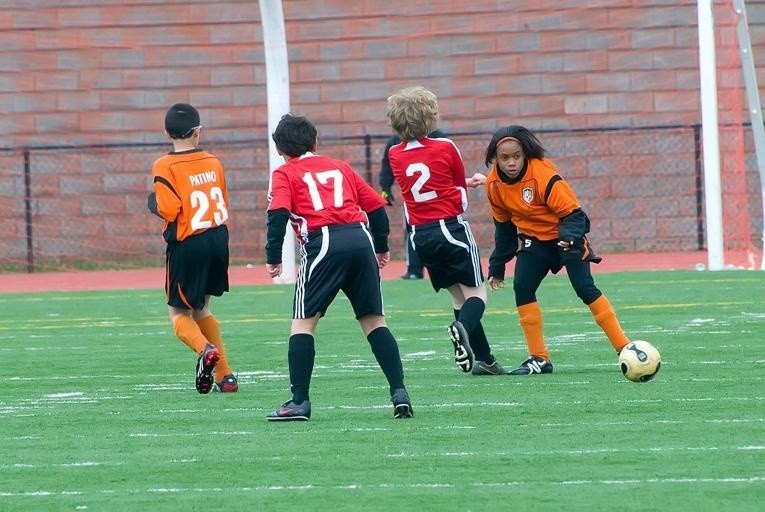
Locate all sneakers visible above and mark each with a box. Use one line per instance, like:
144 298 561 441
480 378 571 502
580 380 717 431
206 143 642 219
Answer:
447 321 552 375
390 388 413 418
266 400 310 420
196 344 237 394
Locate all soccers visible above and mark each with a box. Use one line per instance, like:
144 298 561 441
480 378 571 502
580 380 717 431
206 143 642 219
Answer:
618 340 661 382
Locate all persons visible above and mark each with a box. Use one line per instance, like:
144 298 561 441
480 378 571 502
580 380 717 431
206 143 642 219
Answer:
388 86 506 376
147 103 238 393
379 130 446 280
487 125 629 375
264 115 414 420
